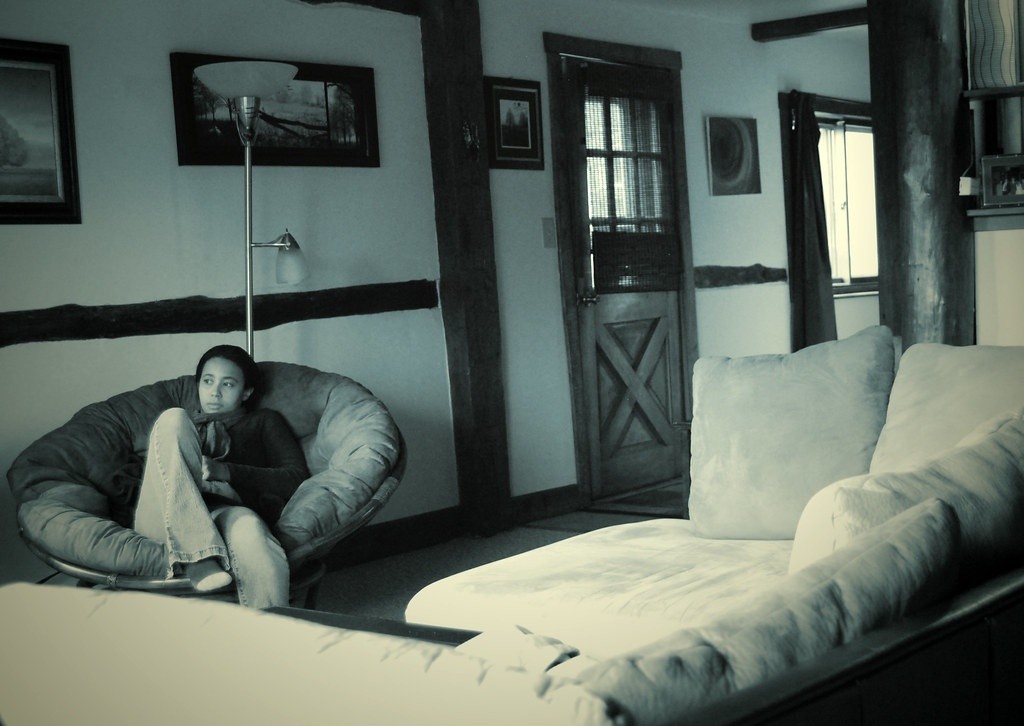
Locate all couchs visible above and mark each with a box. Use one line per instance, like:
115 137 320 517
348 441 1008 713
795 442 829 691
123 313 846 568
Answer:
0 322 1024 726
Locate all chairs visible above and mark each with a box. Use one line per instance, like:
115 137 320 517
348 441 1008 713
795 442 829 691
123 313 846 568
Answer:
7 365 406 586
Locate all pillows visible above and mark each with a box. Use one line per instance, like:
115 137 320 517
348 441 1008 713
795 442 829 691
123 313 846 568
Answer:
789 418 1024 573
688 327 896 535
871 342 1024 478
585 499 952 726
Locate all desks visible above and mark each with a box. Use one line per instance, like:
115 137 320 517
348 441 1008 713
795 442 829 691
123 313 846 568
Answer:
966 208 1024 345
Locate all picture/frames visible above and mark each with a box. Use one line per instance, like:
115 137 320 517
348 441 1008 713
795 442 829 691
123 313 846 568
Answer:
981 154 1023 206
0 37 84 227
169 51 380 167
482 74 545 171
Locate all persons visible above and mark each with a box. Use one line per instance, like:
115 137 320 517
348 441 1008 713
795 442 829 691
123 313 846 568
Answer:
134 346 311 609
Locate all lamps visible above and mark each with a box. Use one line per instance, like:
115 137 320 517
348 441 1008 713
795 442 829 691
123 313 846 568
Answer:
191 62 304 364
